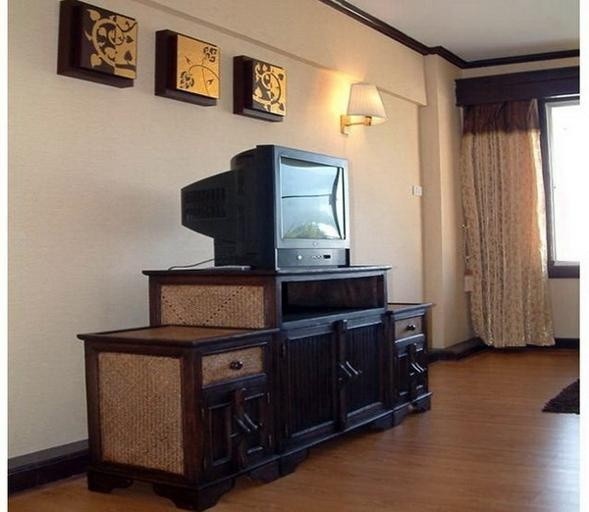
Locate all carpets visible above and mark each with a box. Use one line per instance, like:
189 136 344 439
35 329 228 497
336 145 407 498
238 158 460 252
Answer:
542 378 580 414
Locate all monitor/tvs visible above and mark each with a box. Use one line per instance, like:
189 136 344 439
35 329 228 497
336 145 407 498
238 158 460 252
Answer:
180 144 350 270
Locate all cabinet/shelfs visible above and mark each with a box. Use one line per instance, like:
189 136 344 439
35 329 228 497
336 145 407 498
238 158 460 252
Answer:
77 266 436 512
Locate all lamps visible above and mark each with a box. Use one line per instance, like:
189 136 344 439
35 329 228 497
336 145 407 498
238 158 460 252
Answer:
340 82 388 136
